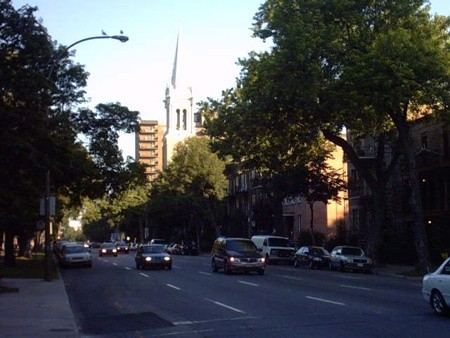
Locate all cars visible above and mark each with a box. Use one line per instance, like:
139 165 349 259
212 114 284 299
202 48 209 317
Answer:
294 245 332 270
90 238 183 257
423 257 450 316
330 245 373 273
135 244 173 270
53 240 92 269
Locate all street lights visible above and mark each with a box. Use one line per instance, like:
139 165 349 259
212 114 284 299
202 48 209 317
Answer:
42 35 130 280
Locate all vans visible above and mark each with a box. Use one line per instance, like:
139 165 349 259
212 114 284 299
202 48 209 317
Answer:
252 235 295 265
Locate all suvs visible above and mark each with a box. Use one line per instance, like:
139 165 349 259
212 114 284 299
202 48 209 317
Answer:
211 235 266 275
